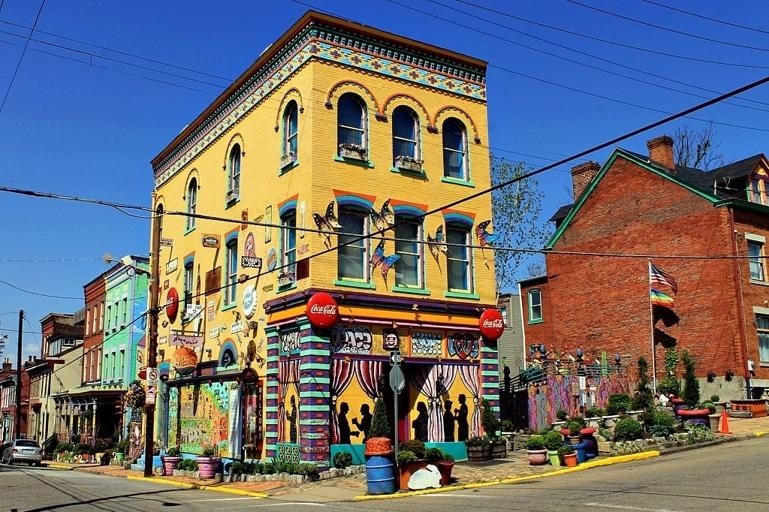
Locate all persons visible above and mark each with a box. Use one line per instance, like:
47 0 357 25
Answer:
652 395 662 406
666 393 675 407
618 408 632 420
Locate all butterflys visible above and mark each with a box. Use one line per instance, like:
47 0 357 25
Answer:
368 239 402 281
475 220 500 246
427 224 448 263
370 198 398 235
312 201 343 239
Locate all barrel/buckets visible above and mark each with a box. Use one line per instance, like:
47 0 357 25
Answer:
365 455 396 495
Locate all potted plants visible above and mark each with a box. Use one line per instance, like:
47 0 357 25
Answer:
704 403 721 433
463 392 515 464
518 411 688 469
389 433 453 494
161 445 223 481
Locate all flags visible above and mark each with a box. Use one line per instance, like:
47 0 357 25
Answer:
650 262 677 309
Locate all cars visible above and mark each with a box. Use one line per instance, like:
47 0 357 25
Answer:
1 439 44 467
